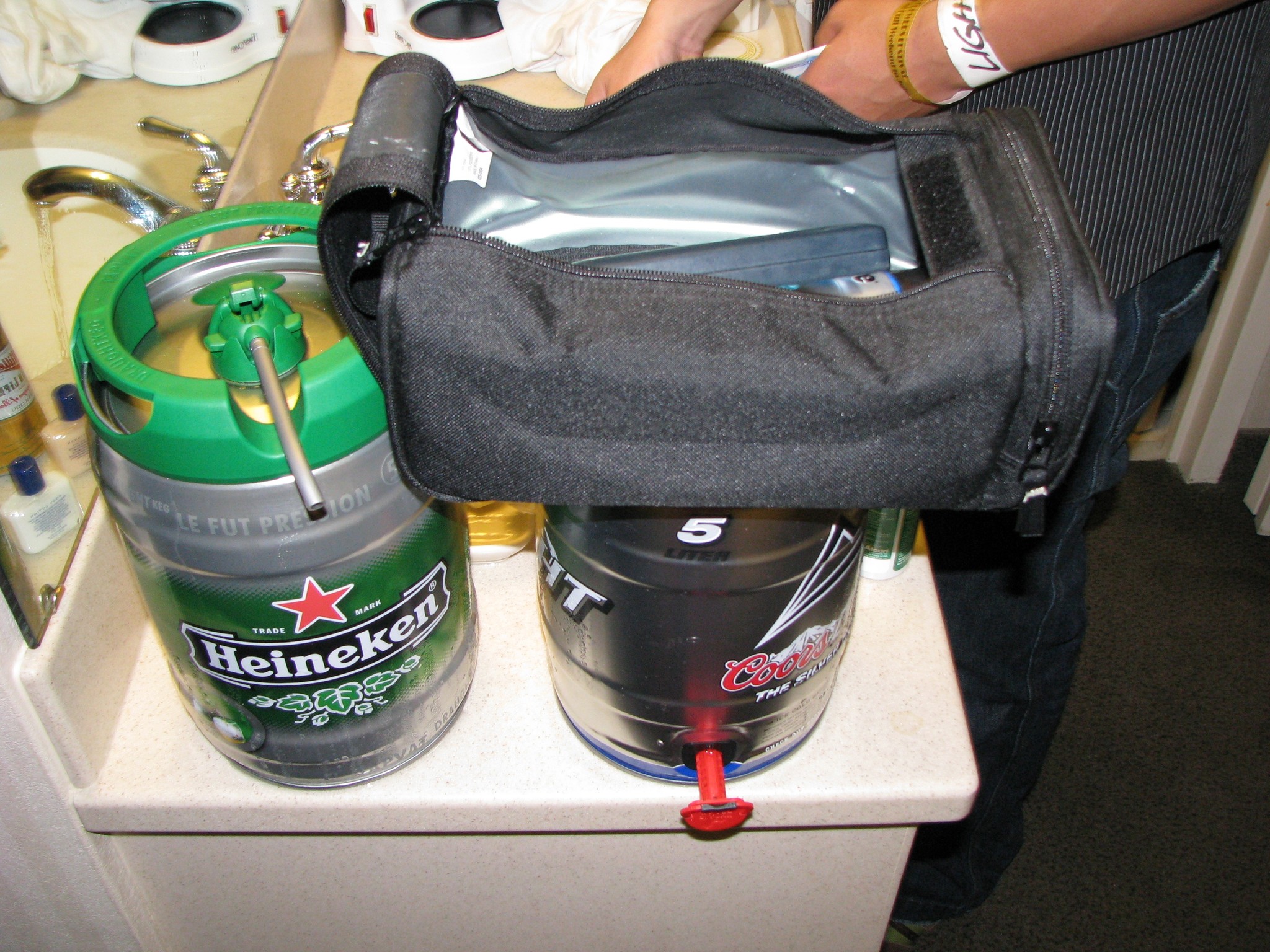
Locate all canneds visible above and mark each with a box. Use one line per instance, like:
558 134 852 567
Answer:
860 504 920 580
0 326 50 477
78 242 477 788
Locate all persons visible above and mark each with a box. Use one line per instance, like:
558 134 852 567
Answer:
582 0 1269 952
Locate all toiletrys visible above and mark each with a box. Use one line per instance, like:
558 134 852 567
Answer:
2 454 86 556
41 382 91 476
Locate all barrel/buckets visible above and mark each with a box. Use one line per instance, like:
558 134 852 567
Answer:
72 203 479 784
537 496 864 831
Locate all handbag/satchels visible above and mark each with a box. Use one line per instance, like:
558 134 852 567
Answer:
314 53 1118 542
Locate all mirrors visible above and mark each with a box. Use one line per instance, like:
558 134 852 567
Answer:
0 0 298 655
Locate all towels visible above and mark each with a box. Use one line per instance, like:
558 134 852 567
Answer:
495 1 647 95
0 0 153 104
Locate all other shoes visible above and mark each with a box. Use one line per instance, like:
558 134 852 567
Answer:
880 920 924 952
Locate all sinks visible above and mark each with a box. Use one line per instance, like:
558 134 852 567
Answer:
3 140 170 380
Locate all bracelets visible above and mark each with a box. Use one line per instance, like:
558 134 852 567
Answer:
887 1 976 108
936 0 1009 89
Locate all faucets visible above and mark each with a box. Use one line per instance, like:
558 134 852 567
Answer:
682 752 755 838
22 115 232 254
197 269 328 517
261 121 359 245
